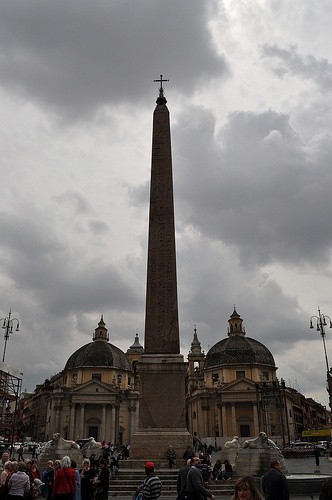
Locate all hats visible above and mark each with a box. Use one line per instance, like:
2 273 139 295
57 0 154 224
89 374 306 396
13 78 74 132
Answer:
143 462 155 469
4 458 90 470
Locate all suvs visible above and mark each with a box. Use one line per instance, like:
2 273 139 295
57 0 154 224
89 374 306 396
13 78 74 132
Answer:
290 442 326 457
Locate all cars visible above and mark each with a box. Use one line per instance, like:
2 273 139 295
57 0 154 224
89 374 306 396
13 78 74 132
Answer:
319 441 332 447
0 438 90 455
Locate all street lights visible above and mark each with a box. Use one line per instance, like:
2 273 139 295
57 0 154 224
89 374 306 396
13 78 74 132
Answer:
309 308 332 392
0 307 19 362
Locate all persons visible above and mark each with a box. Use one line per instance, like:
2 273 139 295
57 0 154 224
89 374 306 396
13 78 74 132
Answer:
262 460 289 500
175 459 193 500
161 427 234 482
186 457 216 499
132 461 162 499
0 435 127 500
230 477 266 500
